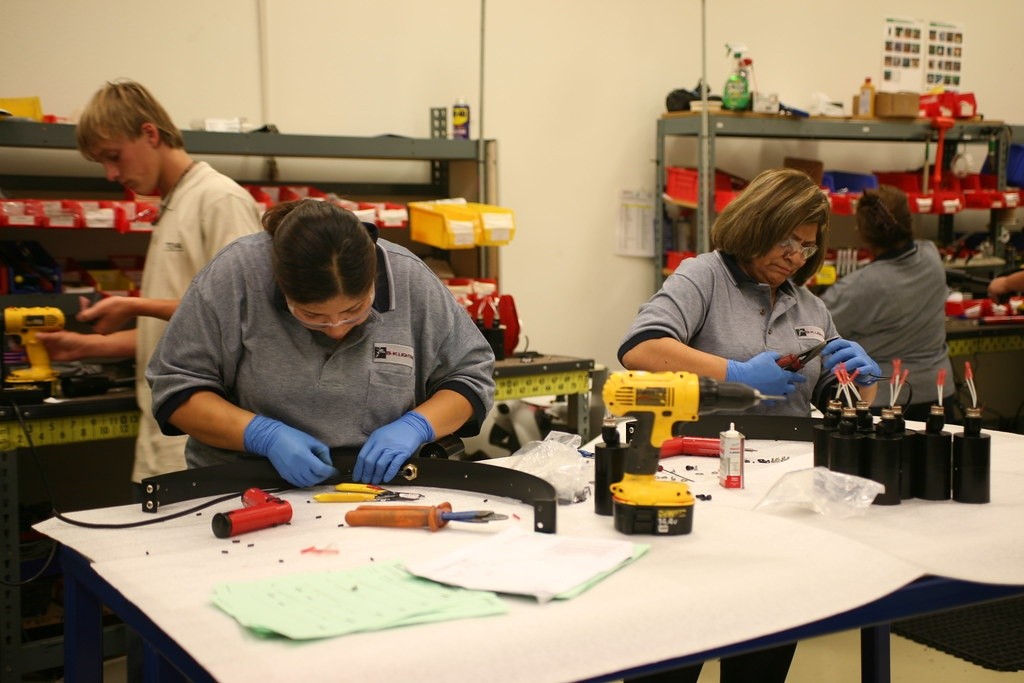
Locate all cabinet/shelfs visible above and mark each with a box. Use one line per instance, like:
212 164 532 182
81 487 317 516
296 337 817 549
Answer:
654 111 1009 303
0 118 500 324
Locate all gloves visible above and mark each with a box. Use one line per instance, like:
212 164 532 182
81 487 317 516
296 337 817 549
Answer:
244 415 337 488
722 351 807 408
351 411 435 486
820 339 882 387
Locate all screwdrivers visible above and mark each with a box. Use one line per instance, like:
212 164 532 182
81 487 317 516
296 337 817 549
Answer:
342 500 509 531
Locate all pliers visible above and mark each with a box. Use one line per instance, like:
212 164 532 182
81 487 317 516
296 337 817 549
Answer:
312 482 426 503
775 334 840 374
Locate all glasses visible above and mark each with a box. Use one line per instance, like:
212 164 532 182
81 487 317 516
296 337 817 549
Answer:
778 237 818 261
285 281 375 331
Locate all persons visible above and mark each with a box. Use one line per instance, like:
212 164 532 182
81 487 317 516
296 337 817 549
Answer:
820 184 956 418
986 270 1024 299
144 198 497 489
33 75 263 682
617 169 882 682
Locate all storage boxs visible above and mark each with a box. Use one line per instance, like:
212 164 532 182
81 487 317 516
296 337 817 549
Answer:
853 92 919 116
0 254 144 313
920 92 977 119
666 144 1024 216
0 184 517 249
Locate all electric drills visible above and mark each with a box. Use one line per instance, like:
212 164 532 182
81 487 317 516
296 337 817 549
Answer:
0 308 66 383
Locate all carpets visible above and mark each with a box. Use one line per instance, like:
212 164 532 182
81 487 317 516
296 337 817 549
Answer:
890 596 1024 672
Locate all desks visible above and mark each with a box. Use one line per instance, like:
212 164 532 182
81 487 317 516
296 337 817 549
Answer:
946 315 1024 435
1 354 595 682
34 414 1024 683
